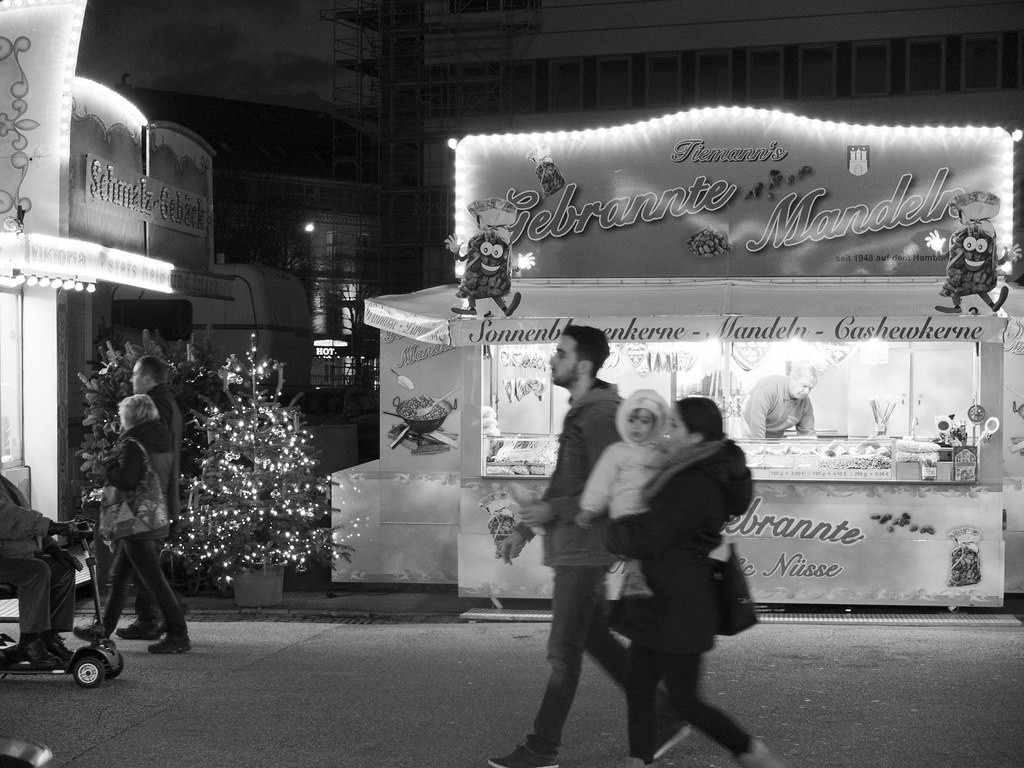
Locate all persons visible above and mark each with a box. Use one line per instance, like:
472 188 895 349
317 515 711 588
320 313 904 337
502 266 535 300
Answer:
116 357 183 641
486 324 692 768
0 473 83 669
74 394 191 653
738 362 818 440
575 387 672 632
605 396 779 768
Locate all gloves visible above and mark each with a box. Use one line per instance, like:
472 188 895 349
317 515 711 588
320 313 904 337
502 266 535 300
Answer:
48 542 84 571
49 519 76 537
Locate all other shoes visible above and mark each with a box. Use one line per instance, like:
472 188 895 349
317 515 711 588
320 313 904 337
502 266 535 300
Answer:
488 741 559 768
650 716 690 761
620 572 653 598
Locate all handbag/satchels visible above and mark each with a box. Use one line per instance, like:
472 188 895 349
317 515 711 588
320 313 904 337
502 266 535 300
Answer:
98 438 168 540
708 541 758 637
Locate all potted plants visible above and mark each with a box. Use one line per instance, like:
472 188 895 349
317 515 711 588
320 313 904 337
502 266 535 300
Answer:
76 325 354 608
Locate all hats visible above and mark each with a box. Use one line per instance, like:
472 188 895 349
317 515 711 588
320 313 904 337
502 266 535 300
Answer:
629 400 661 436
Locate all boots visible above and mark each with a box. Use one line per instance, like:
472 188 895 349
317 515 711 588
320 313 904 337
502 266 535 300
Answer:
622 756 658 768
736 737 790 768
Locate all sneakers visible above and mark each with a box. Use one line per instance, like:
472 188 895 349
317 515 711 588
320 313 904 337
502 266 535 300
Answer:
147 631 191 653
74 623 110 643
43 633 74 660
15 636 61 666
115 618 160 640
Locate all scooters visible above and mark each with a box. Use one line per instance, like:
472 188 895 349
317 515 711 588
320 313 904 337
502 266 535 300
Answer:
0 520 126 689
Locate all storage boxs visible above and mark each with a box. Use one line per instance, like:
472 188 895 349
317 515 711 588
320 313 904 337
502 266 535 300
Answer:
896 462 920 480
936 462 953 481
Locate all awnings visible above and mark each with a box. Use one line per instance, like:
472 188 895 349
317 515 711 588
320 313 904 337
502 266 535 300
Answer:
362 281 1011 346
